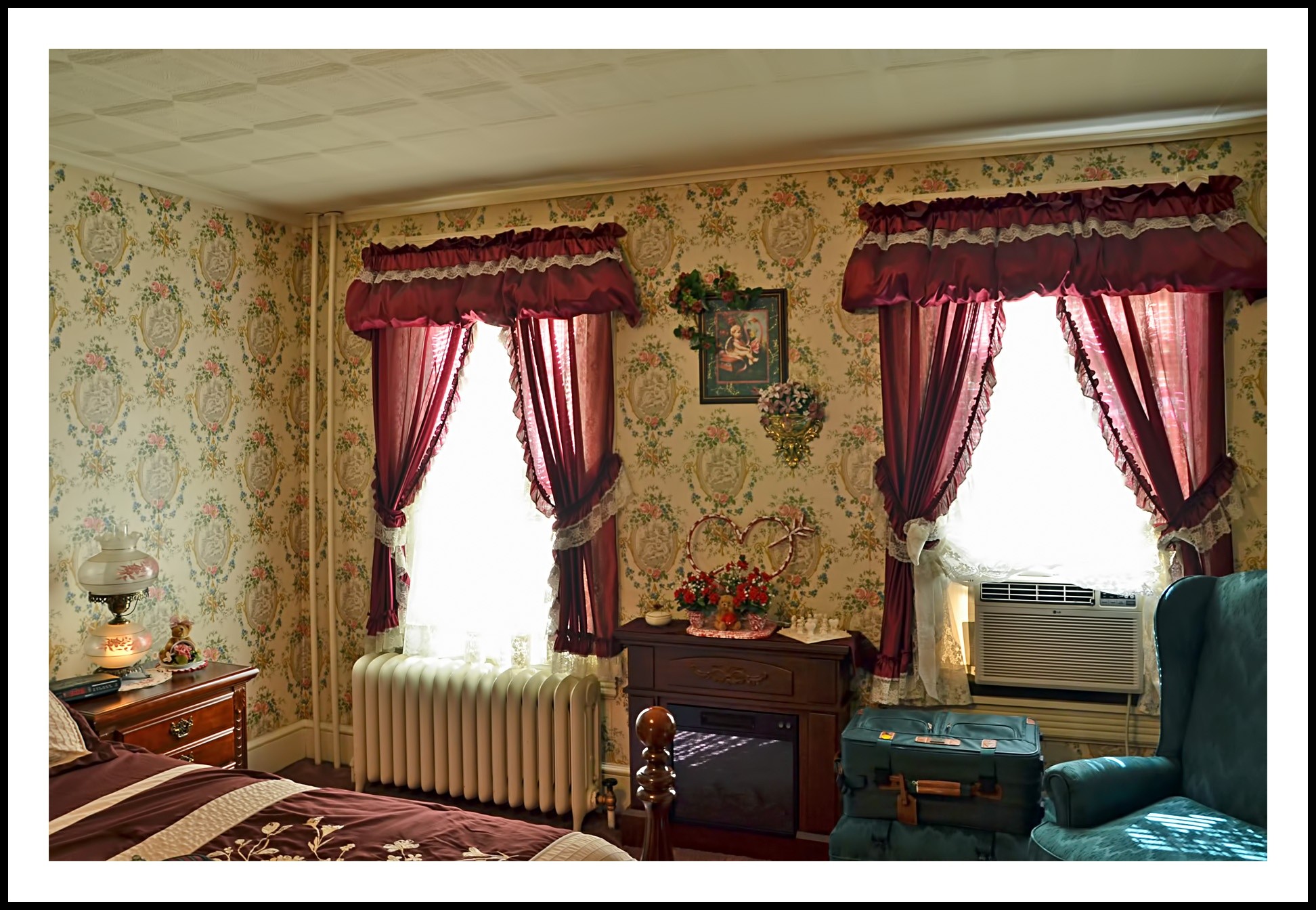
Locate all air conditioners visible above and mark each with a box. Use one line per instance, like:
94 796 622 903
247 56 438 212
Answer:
973 578 1145 695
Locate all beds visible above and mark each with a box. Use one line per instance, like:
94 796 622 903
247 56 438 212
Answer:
49 741 637 862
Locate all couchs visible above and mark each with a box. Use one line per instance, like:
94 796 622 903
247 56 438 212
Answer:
1029 565 1267 861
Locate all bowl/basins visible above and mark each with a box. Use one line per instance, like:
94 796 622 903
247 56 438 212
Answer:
645 610 671 626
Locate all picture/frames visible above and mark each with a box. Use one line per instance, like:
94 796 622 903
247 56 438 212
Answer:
699 288 788 404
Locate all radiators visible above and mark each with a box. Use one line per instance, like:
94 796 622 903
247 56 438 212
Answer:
349 650 615 834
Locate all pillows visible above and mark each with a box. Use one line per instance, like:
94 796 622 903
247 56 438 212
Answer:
49 689 119 777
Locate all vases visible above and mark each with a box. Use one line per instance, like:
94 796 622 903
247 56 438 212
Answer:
748 610 767 631
685 611 705 629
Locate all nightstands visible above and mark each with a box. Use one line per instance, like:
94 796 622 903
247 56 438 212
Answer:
65 660 260 771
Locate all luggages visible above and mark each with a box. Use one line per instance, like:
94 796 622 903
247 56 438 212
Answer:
832 707 1046 836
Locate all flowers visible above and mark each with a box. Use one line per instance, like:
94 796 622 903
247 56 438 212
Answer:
672 558 776 615
669 269 764 353
755 380 820 425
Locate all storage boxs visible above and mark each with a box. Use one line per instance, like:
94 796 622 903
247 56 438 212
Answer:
831 706 1044 861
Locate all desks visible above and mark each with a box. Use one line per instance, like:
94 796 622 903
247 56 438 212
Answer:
615 618 862 861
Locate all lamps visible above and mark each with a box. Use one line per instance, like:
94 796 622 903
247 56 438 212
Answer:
79 530 160 680
